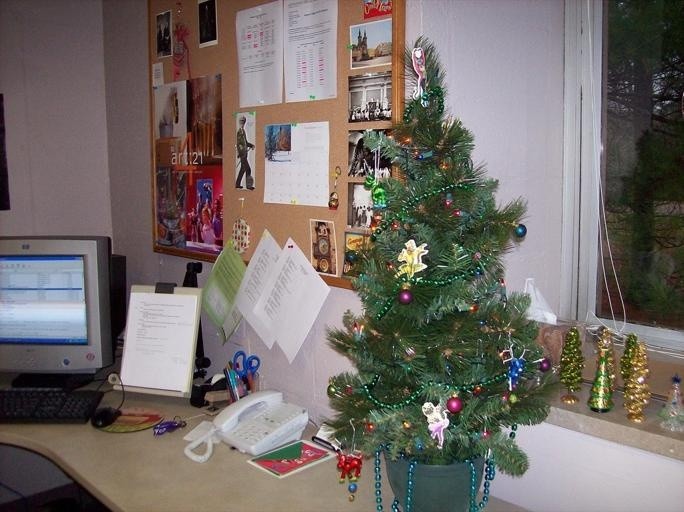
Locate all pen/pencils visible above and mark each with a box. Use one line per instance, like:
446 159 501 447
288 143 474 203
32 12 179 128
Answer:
219 361 246 399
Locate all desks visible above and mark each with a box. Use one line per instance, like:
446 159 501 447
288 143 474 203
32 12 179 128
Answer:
0 374 530 512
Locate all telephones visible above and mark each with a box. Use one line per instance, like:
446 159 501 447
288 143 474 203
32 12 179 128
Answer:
211 388 308 457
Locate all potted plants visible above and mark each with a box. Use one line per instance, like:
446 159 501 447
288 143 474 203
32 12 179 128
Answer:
328 38 553 511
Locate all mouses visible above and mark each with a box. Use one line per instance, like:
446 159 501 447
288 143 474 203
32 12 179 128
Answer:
91 408 121 428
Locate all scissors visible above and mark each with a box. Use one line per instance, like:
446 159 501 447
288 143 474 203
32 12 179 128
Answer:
233 350 260 376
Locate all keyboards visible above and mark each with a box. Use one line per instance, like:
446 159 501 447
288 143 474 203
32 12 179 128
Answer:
0 388 104 425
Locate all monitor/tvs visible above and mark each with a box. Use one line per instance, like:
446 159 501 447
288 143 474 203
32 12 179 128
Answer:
0 236 126 388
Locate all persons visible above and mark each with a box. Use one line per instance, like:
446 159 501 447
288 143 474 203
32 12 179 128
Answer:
164 21 170 51
188 192 223 244
352 104 392 121
356 205 373 227
236 116 255 190
158 23 164 57
